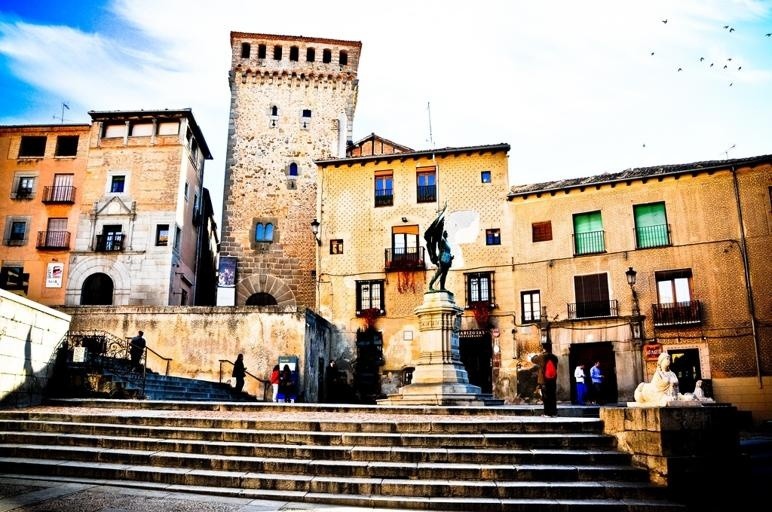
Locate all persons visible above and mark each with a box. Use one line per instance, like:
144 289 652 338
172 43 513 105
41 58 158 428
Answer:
232 354 248 392
651 352 680 401
574 361 588 405
536 343 558 417
325 359 338 403
271 365 280 402
429 230 454 294
281 365 292 403
590 359 606 405
129 331 146 374
